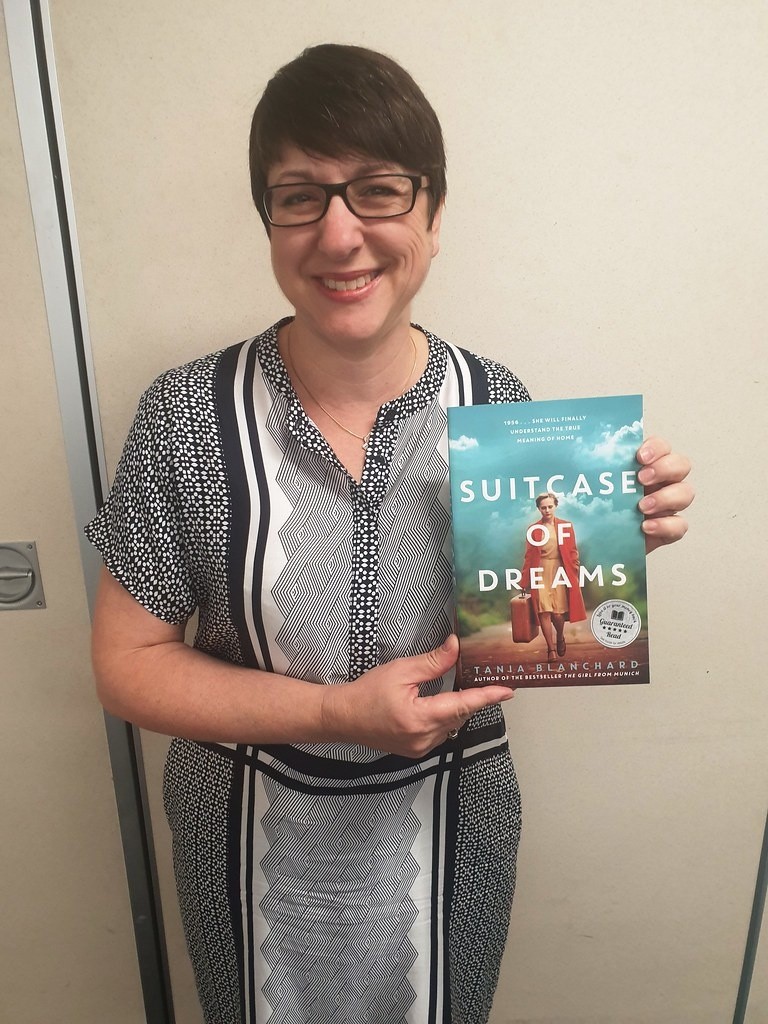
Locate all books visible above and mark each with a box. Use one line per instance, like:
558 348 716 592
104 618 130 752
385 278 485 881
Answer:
447 394 650 690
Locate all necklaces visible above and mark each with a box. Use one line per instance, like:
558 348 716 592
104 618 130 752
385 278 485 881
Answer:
288 324 417 450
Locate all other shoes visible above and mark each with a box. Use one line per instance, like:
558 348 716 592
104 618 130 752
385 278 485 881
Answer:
546 649 558 663
557 635 566 657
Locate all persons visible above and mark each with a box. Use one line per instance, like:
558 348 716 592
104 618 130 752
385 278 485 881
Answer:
520 494 586 664
84 43 694 1024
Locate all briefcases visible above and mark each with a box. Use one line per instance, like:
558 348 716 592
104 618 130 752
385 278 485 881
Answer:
509 588 540 643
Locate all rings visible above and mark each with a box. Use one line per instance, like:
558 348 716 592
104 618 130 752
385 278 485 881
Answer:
449 729 458 738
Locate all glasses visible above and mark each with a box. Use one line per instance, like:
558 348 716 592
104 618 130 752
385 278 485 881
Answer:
261 173 430 228
